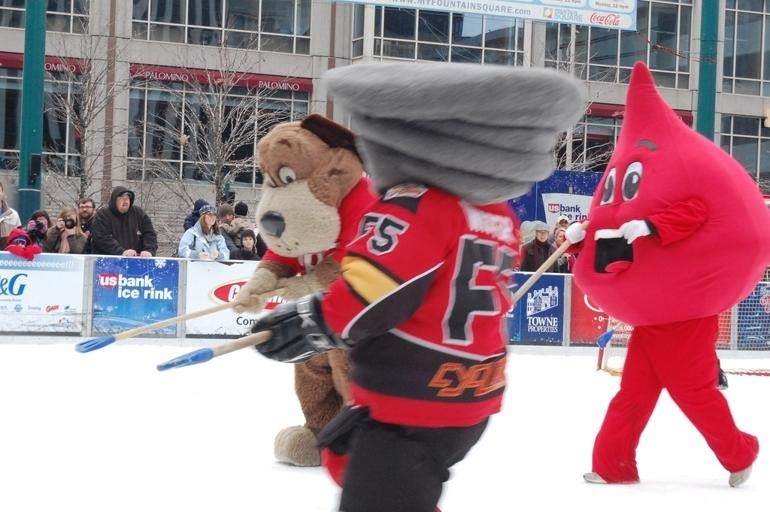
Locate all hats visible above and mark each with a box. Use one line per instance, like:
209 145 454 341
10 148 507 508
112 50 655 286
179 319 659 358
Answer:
235 201 247 215
536 222 549 232
13 236 26 245
199 205 216 215
221 204 233 217
556 215 569 226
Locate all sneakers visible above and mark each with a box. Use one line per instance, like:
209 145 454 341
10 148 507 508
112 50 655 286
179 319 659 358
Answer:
583 471 606 483
728 464 752 487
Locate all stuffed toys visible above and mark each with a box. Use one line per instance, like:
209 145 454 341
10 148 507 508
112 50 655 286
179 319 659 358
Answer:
232 111 381 469
555 59 770 490
249 56 588 512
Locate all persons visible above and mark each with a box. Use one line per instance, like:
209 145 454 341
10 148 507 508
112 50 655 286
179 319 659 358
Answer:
520 223 559 273
548 212 570 244
551 227 577 273
1 181 268 263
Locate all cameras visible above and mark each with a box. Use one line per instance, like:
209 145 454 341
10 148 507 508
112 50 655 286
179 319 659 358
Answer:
36 221 44 230
64 216 73 229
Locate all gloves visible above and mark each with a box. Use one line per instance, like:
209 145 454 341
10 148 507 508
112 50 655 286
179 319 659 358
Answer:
619 220 650 244
564 222 586 244
